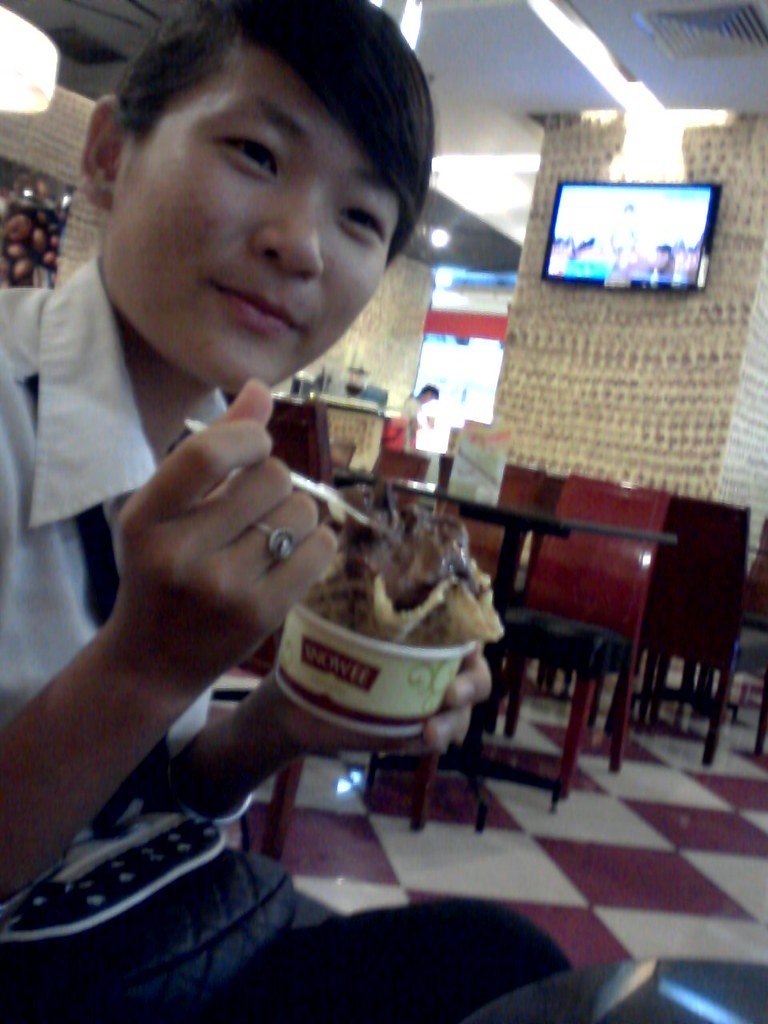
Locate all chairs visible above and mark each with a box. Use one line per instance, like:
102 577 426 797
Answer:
224 389 768 862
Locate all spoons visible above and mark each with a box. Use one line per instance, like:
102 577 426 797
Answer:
183 419 371 528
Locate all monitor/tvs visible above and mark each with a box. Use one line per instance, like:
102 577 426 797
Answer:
542 181 723 291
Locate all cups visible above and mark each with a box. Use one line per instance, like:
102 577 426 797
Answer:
290 370 314 404
271 601 476 739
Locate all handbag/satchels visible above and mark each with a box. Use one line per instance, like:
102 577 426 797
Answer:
0 807 298 1019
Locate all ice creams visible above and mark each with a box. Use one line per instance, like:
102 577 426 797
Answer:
297 482 504 652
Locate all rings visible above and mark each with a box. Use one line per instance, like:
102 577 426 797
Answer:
255 523 294 560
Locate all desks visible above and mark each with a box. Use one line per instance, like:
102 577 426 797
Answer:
337 466 677 832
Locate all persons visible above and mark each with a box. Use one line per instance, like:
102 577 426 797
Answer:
1 0 571 1023
401 385 438 429
